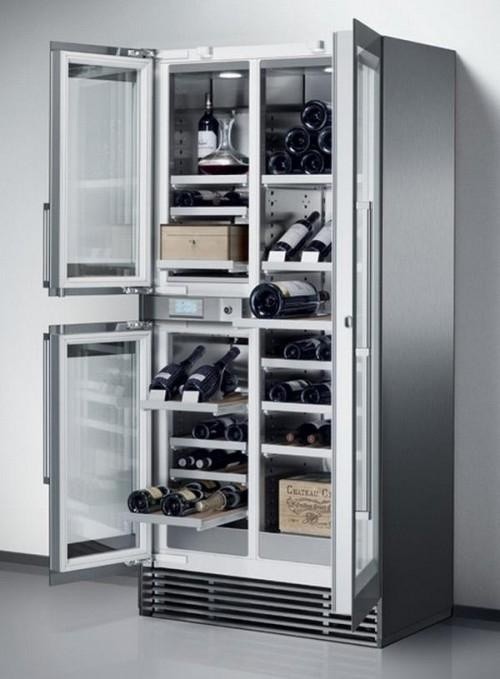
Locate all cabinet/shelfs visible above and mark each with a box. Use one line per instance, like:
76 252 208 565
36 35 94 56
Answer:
42 18 458 650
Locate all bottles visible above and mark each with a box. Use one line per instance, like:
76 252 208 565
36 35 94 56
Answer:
308 426 332 449
127 485 178 514
149 345 206 400
284 335 324 360
196 483 248 512
219 190 249 206
225 423 248 442
183 348 241 402
318 127 332 153
302 148 331 175
267 150 303 175
301 383 331 404
175 191 223 205
250 281 329 318
198 118 249 174
301 98 333 131
285 128 316 158
196 449 248 471
305 219 332 260
315 340 330 358
192 418 241 439
187 482 220 495
287 422 326 446
271 211 319 259
198 92 218 173
161 488 215 516
270 379 310 401
178 450 214 469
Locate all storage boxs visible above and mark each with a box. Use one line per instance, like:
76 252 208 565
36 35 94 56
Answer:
277 472 331 537
159 222 245 260
